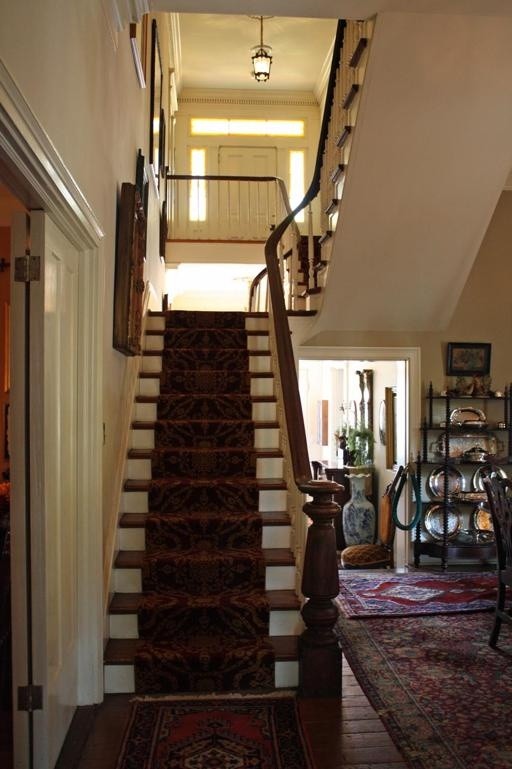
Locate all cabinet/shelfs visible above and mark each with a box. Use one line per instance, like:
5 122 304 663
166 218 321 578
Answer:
412 380 512 569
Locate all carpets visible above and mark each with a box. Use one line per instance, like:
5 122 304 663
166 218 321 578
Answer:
335 613 512 769
330 569 512 619
115 689 318 769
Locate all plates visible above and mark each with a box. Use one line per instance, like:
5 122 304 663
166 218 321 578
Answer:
429 464 465 498
424 502 463 543
473 464 508 495
473 509 494 532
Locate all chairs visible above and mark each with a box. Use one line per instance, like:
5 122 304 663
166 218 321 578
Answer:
340 483 394 569
480 472 512 649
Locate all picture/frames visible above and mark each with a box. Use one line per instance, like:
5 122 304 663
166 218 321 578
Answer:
446 342 492 376
112 13 168 357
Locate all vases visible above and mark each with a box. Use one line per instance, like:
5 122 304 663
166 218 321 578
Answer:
342 474 376 547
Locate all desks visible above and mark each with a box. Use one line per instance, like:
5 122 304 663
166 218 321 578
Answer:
324 467 349 550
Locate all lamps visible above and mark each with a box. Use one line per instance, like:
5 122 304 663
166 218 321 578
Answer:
247 15 275 83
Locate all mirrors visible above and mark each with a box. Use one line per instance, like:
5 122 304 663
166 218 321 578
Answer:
356 369 373 464
